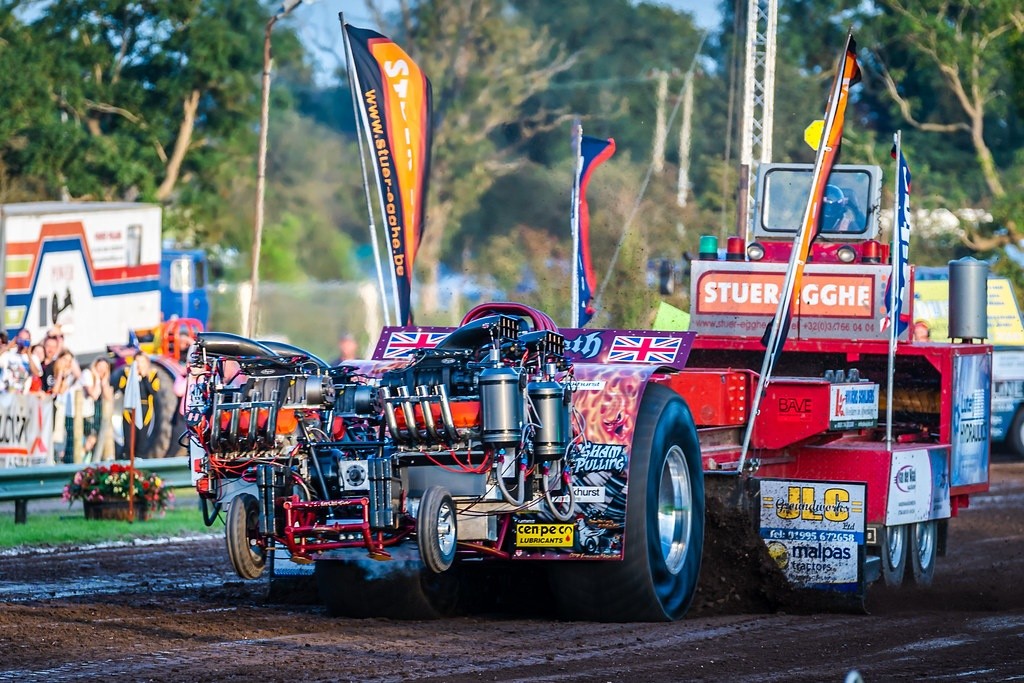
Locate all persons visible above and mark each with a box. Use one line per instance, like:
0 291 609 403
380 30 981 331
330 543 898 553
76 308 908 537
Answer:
818 183 866 233
0 326 249 468
914 319 934 343
330 327 366 367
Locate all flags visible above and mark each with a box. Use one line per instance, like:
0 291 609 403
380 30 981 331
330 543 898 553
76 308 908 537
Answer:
879 142 912 357
760 35 863 400
341 23 435 328
566 132 617 328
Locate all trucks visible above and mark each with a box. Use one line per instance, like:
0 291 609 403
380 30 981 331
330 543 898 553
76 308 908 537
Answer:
1 199 209 367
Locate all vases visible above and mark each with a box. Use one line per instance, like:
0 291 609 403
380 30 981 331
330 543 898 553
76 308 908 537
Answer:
82 492 148 522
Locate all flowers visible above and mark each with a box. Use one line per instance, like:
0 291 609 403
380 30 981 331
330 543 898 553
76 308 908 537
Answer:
60 461 173 520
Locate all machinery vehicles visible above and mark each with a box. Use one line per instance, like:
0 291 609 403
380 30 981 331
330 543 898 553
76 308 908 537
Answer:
177 1 996 622
